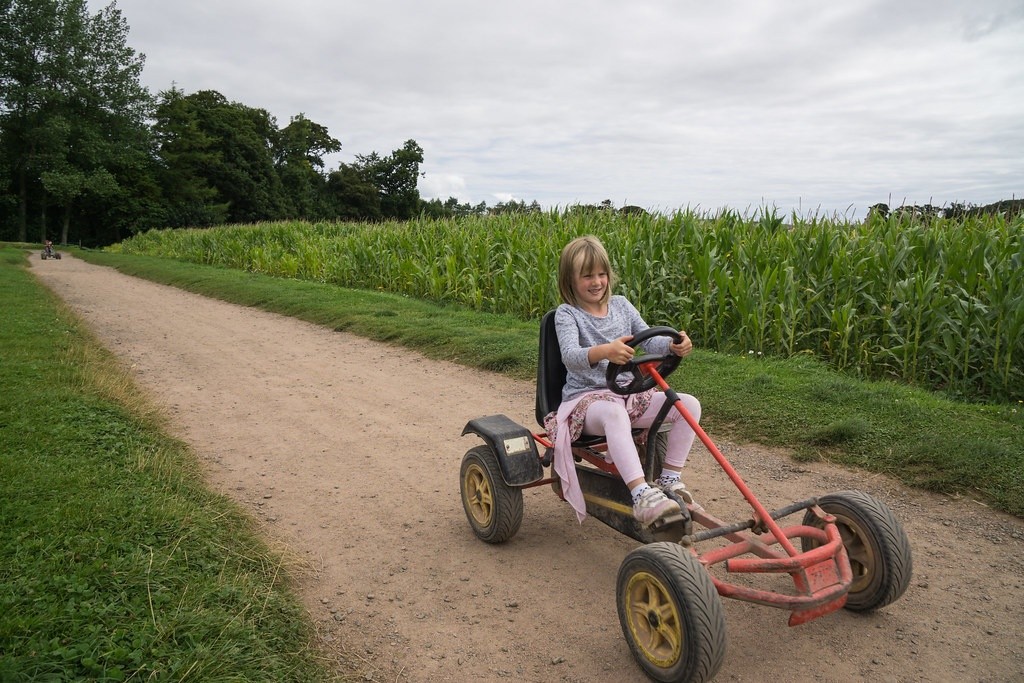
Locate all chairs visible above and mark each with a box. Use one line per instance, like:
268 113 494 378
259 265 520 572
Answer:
534 309 644 443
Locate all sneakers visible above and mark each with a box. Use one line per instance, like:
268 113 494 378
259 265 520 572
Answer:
633 488 680 528
656 478 704 512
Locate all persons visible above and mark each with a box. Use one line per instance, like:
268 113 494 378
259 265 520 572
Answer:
44 240 51 254
543 235 700 527
